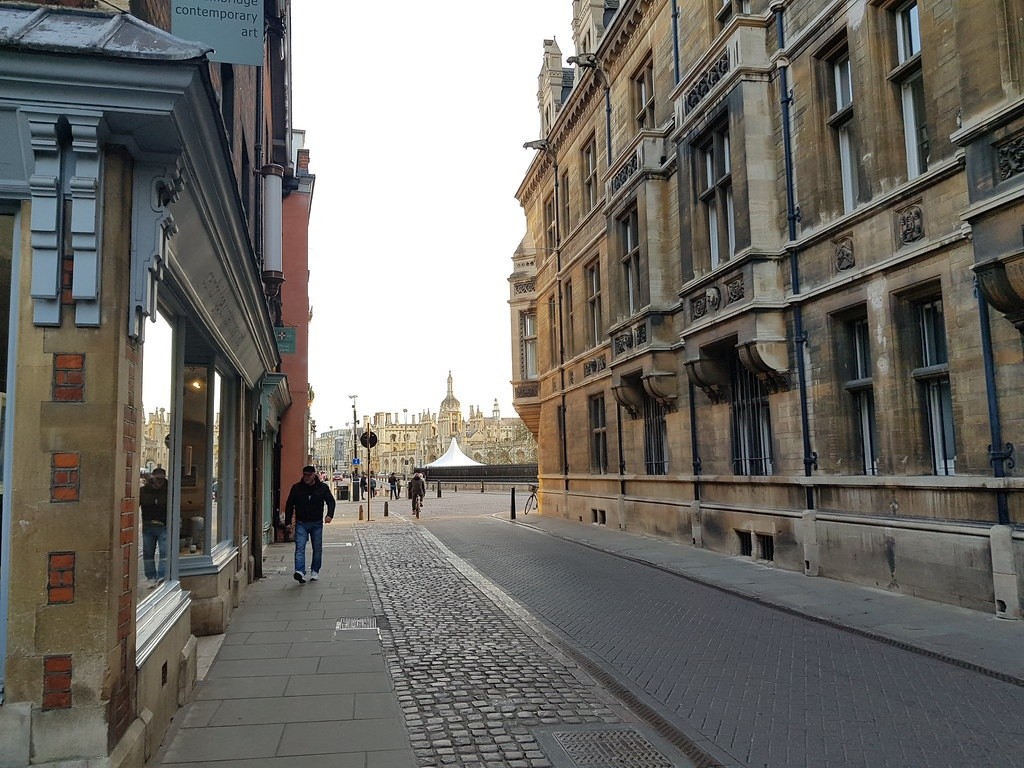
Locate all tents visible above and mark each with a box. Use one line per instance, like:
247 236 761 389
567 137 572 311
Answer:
424 437 486 467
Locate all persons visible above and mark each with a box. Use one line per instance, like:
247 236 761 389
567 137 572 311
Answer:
397 479 401 498
408 473 425 515
419 473 426 499
370 475 376 497
285 466 336 583
360 475 367 500
140 468 168 589
318 471 328 482
388 472 399 499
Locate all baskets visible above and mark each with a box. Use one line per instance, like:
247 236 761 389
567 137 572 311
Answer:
529 485 539 492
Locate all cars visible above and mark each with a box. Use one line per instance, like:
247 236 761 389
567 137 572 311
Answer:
332 469 350 482
317 470 329 483
140 468 151 478
379 471 388 476
212 481 220 492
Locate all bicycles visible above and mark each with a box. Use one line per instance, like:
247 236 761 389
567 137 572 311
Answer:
409 490 425 519
525 484 539 515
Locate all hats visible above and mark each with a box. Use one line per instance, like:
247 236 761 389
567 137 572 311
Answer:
303 466 315 475
151 468 165 479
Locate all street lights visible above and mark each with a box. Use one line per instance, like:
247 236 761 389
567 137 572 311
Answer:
349 395 360 480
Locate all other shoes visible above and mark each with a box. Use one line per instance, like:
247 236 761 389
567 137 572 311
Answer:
412 512 415 515
396 497 399 499
420 503 423 507
362 498 366 500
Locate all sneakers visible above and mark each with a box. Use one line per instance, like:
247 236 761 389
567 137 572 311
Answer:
147 578 156 588
309 571 319 581
294 570 306 583
